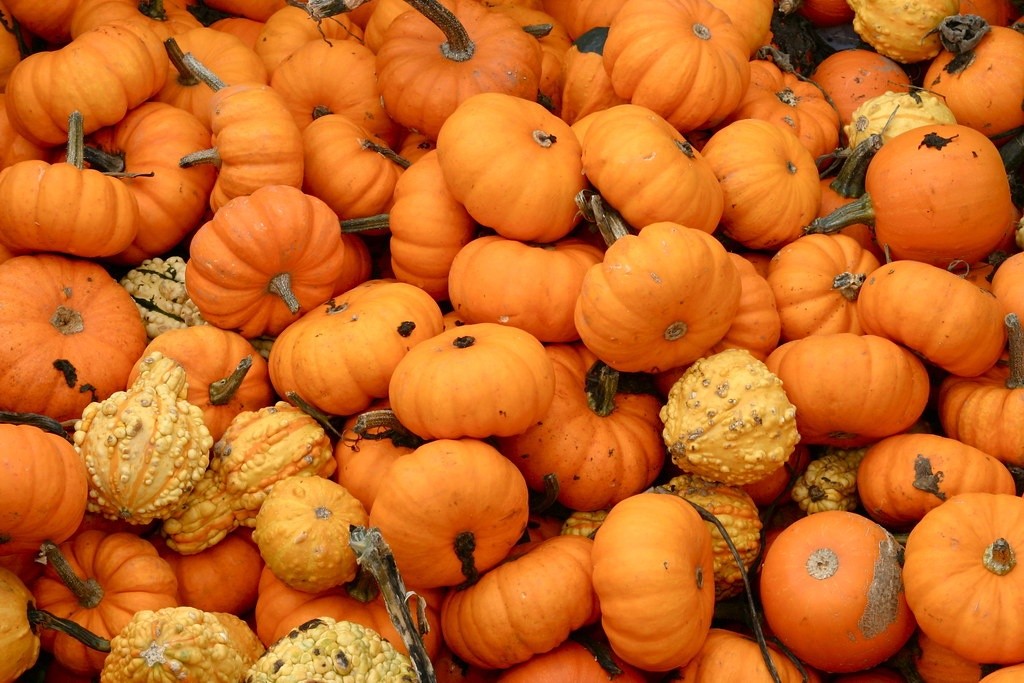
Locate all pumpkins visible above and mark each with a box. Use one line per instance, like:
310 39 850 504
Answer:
0 0 1024 683
